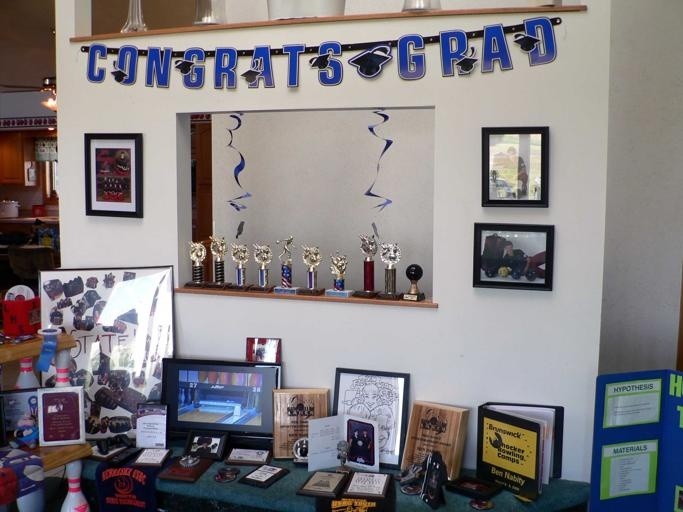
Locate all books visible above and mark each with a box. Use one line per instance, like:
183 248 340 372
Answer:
477 402 565 502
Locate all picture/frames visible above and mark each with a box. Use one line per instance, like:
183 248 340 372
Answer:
470 221 556 290
341 471 390 499
35 385 86 446
226 446 270 468
37 264 176 442
183 429 225 461
130 448 170 467
332 367 411 470
85 134 145 221
296 469 346 499
237 465 289 489
477 124 551 207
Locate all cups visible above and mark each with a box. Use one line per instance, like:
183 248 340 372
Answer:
31 205 45 218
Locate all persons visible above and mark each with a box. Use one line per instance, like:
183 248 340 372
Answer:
349 429 372 461
504 147 528 194
502 241 545 278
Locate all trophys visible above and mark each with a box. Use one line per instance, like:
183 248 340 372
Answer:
325 248 354 298
376 242 404 301
231 243 254 292
400 264 425 302
184 240 209 289
298 245 326 296
273 235 301 295
208 236 232 290
248 243 276 293
353 233 381 299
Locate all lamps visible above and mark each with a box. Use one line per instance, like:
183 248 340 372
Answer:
38 79 56 113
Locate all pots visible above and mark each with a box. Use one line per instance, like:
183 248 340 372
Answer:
0 200 19 219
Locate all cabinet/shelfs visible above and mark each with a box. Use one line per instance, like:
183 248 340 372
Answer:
0 335 93 511
0 131 40 188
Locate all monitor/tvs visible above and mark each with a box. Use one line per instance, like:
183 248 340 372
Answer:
161 357 282 440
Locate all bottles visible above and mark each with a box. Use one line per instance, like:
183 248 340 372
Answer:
53 351 74 388
59 458 90 512
14 356 39 388
35 329 60 374
